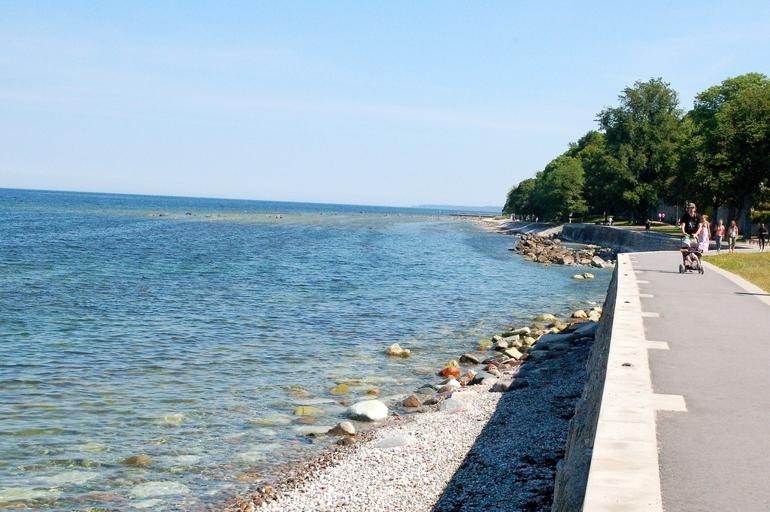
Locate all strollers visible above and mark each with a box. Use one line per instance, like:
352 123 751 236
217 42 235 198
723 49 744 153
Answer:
679 234 704 274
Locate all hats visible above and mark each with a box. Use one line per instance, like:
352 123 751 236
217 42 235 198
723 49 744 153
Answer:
688 202 696 208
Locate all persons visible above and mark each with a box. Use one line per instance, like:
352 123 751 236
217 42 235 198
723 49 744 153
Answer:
645 218 651 234
680 203 739 265
758 223 768 252
657 211 666 222
